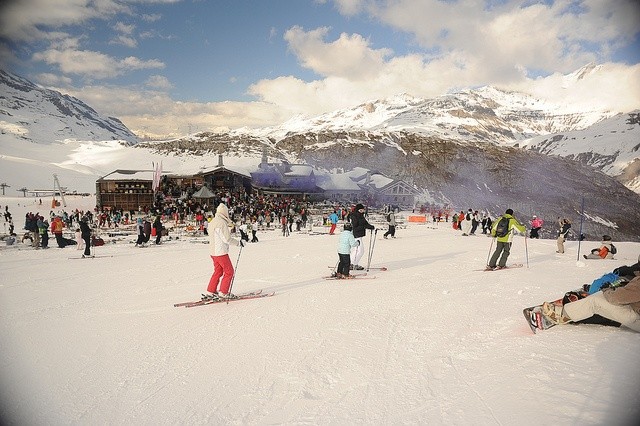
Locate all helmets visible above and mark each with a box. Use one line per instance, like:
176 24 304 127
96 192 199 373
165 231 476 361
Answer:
344 222 353 232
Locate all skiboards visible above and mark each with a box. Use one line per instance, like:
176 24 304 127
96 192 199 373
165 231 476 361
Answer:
174 290 275 308
322 273 376 280
472 264 523 272
68 256 113 259
328 266 387 271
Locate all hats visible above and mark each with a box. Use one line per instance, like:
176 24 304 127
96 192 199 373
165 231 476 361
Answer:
83 217 89 223
356 203 364 212
334 211 336 213
217 203 228 218
602 235 612 241
39 216 44 220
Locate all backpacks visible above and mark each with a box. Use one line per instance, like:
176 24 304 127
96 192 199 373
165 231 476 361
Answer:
496 216 515 237
387 213 395 222
538 219 544 225
608 243 617 254
466 212 472 221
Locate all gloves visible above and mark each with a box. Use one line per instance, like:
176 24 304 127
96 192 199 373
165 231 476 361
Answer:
591 248 598 252
239 239 246 247
592 250 596 254
358 240 360 246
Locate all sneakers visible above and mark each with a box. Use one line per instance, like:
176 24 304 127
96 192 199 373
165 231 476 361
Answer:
219 291 234 298
135 243 138 246
462 233 465 236
84 254 86 257
536 235 539 239
487 265 496 269
138 244 142 247
530 237 533 239
85 255 93 258
383 235 388 239
498 264 508 268
330 233 335 235
156 242 162 245
353 265 364 270
336 273 342 277
543 302 571 325
392 236 396 238
465 233 468 236
350 264 354 270
47 246 50 248
43 247 48 249
342 274 352 279
556 251 564 253
206 290 219 298
583 255 587 259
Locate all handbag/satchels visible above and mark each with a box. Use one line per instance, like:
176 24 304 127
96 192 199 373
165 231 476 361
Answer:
152 228 157 236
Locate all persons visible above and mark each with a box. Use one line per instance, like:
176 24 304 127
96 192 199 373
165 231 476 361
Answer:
216 188 236 203
69 209 74 226
281 196 308 236
80 216 94 257
266 214 271 227
239 221 248 246
32 215 40 247
75 229 84 250
337 223 360 279
458 210 465 230
142 218 151 245
329 211 338 235
51 217 65 248
488 209 527 270
487 217 492 234
584 235 612 259
481 215 487 234
75 209 79 222
270 197 281 223
186 199 194 221
166 201 186 224
229 204 244 220
531 215 542 238
194 201 211 236
383 210 397 239
347 204 377 270
246 197 270 213
365 207 370 217
258 214 264 225
207 203 246 299
99 205 135 227
544 255 640 332
336 205 350 222
135 218 146 247
453 214 458 230
463 208 473 236
154 216 164 245
37 216 49 249
138 201 166 214
556 219 571 253
252 219 258 242
251 215 257 242
580 233 585 240
469 211 480 235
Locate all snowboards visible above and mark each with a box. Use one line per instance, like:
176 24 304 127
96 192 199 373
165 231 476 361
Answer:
523 299 563 334
